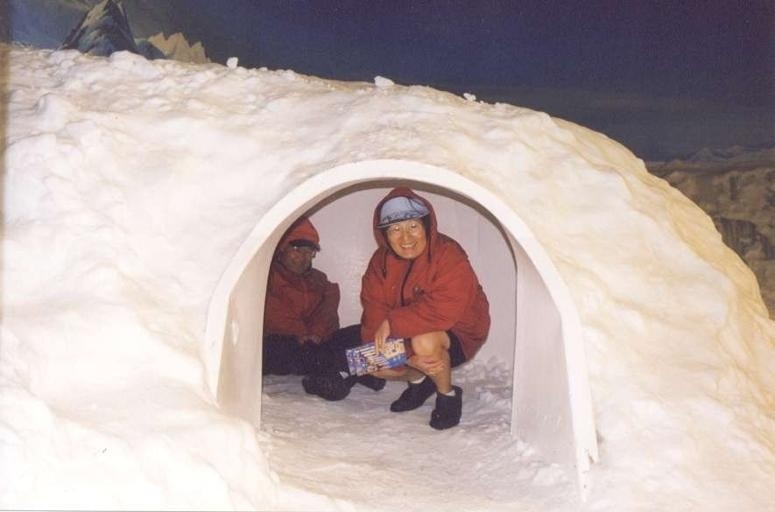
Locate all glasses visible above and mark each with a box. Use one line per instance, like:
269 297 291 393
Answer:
296 249 315 258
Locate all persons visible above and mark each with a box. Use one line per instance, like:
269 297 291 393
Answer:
360 188 490 430
262 217 386 391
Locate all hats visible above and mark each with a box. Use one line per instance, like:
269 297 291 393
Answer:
376 196 430 229
289 240 315 249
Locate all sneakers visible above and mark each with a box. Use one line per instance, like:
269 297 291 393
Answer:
390 375 437 413
303 371 350 401
429 385 463 430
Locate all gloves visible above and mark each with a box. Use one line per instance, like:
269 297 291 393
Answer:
301 339 329 373
